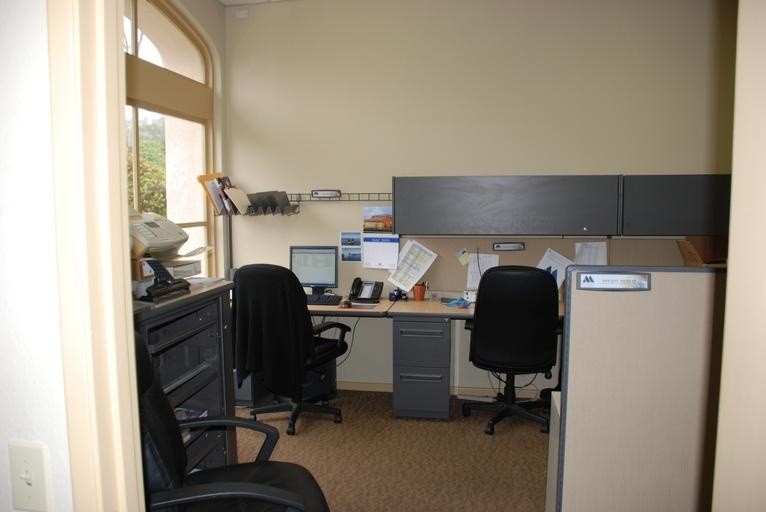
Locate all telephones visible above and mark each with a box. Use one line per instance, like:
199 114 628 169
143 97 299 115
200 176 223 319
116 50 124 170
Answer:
348 278 383 303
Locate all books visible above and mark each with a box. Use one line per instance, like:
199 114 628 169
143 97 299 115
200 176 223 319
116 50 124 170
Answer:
198 171 237 216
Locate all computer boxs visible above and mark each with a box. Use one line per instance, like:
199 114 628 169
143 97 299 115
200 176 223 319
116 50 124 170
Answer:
273 359 336 402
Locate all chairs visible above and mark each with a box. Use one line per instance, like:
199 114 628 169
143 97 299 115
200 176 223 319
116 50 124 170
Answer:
460 265 560 437
134 329 332 512
232 265 352 436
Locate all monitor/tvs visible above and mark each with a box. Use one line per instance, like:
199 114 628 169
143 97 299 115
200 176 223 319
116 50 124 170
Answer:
289 246 338 294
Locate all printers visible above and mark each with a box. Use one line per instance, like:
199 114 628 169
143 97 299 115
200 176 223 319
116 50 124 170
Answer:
128 206 213 281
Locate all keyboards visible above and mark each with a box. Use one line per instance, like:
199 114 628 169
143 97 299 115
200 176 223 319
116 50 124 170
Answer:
306 294 342 305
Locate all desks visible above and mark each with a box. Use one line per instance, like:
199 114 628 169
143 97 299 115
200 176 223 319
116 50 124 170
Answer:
386 300 566 422
226 292 395 409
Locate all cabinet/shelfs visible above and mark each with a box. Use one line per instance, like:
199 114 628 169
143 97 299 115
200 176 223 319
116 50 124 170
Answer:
130 273 237 473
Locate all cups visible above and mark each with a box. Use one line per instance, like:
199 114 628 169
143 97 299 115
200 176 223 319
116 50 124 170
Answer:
413 284 425 301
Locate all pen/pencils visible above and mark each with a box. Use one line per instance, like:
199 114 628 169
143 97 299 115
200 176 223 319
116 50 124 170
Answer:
421 280 429 288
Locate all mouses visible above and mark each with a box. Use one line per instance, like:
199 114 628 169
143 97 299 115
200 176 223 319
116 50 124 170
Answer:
342 301 351 307
